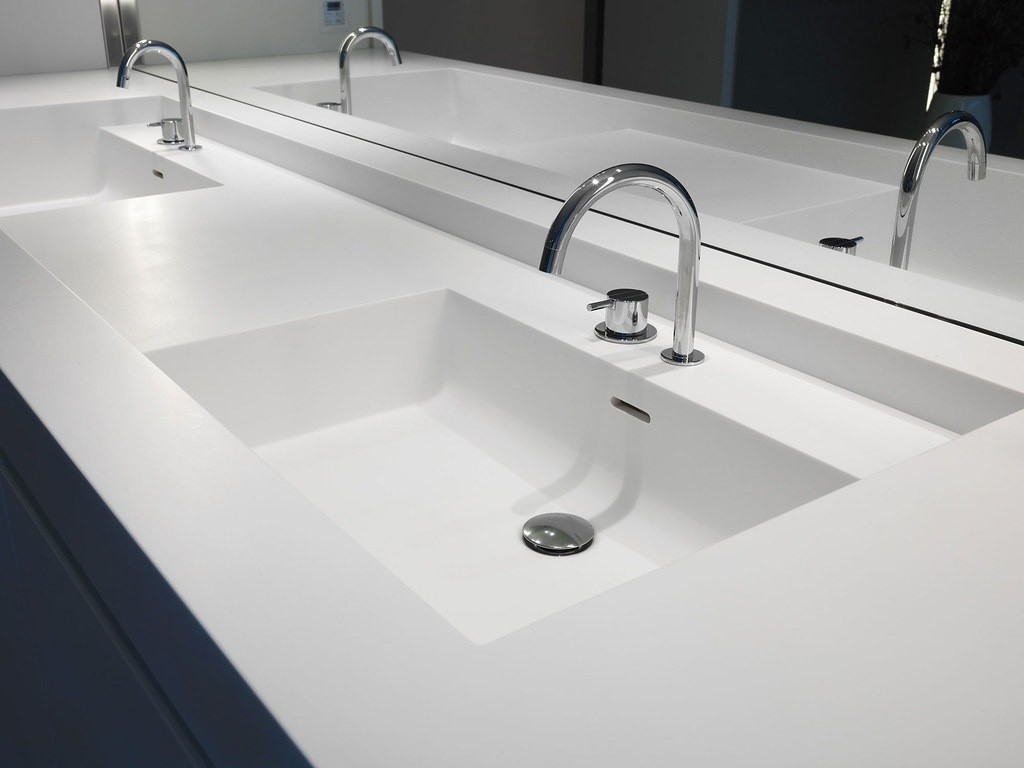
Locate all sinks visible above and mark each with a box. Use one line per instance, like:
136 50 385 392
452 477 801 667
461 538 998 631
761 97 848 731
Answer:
249 68 630 149
740 187 1024 306
140 284 864 649
0 127 226 217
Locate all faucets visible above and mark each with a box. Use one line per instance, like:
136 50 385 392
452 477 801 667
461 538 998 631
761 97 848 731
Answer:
115 38 203 151
536 162 706 366
336 25 402 116
888 111 987 271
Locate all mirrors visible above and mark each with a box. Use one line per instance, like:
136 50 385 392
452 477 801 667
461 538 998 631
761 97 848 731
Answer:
116 0 1024 343
